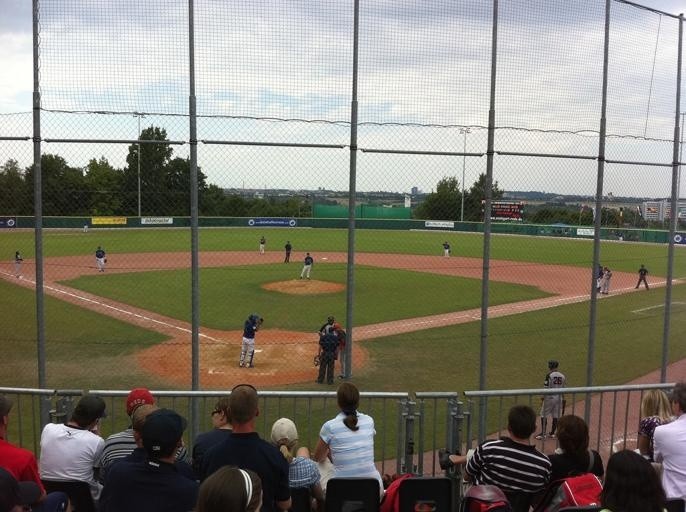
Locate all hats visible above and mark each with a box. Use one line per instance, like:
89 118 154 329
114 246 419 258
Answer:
75 395 108 419
333 323 340 330
270 417 298 447
0 392 14 419
126 388 153 414
141 407 188 442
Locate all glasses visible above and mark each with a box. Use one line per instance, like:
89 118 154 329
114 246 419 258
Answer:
211 410 220 416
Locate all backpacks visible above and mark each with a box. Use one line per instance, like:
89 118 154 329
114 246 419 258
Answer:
533 448 606 512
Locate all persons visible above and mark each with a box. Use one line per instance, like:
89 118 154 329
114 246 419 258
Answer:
271 418 321 512
533 416 604 512
102 404 197 483
97 410 198 512
464 405 546 512
653 383 686 512
595 265 603 293
0 393 70 512
314 382 379 503
531 361 565 441
600 450 666 512
444 242 449 256
95 246 106 272
84 221 89 233
197 467 261 511
199 385 291 512
637 389 675 463
14 251 21 277
239 315 263 369
96 387 191 486
635 264 648 290
315 327 339 386
437 449 475 470
333 324 352 377
39 394 106 504
300 253 313 280
284 241 292 263
260 236 266 255
600 266 612 294
188 398 232 477
318 317 336 337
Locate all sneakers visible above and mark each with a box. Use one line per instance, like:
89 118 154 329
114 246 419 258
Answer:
535 433 546 440
547 432 555 438
438 448 454 470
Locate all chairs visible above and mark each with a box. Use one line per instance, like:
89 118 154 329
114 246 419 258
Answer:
398 477 454 512
39 478 96 512
558 505 600 512
287 486 313 512
324 477 380 512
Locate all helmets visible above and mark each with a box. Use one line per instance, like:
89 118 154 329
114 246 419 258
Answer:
327 316 334 325
249 314 258 320
548 359 558 369
